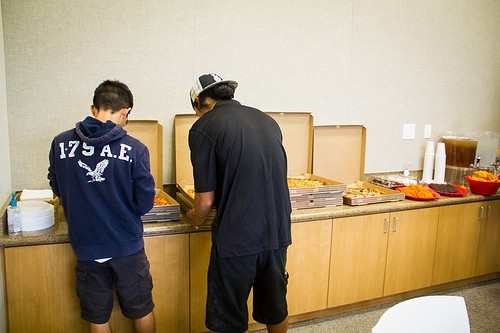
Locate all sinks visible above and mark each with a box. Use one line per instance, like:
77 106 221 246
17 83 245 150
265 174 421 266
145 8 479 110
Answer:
402 167 475 184
461 167 500 192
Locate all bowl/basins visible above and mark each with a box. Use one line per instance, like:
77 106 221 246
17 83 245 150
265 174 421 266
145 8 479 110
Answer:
465 176 500 196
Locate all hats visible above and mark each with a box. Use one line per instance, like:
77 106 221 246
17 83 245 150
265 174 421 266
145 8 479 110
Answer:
189 72 238 109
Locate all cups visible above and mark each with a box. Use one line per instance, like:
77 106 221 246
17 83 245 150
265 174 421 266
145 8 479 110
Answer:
421 141 446 182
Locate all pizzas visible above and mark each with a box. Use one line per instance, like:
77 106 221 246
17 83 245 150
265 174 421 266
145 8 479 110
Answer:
287 176 331 188
154 196 170 206
343 187 388 198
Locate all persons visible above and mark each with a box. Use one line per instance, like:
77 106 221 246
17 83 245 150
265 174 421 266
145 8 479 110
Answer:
47 79 155 333
186 73 292 333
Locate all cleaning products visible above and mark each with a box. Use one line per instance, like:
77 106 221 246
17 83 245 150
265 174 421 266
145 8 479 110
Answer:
6 192 23 239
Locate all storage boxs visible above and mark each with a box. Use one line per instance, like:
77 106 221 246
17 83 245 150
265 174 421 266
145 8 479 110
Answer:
123 109 404 223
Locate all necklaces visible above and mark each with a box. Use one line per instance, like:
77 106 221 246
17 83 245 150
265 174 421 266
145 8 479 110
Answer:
211 102 217 110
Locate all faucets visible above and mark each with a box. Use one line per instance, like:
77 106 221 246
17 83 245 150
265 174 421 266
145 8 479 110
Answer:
489 154 500 169
469 155 481 169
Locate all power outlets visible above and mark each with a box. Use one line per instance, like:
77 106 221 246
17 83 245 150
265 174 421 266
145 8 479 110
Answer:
424 123 431 139
404 123 416 140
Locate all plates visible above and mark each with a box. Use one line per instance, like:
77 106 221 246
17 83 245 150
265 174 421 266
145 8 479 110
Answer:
7 200 56 232
395 186 439 200
426 183 468 196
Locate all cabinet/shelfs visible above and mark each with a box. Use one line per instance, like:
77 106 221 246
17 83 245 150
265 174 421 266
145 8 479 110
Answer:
5 234 190 333
189 219 335 333
433 201 500 283
328 207 441 309
